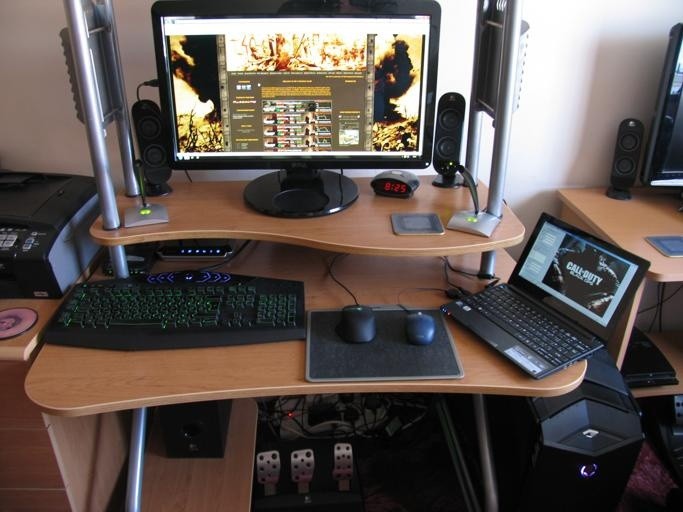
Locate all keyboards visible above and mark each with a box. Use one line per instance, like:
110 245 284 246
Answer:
37 269 308 352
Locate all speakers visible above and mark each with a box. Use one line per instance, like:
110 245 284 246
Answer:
606 120 644 200
131 99 172 198
431 91 466 190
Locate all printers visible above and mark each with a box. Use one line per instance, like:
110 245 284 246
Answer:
0 169 104 299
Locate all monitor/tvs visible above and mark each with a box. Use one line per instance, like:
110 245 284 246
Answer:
151 0 442 220
639 23 683 189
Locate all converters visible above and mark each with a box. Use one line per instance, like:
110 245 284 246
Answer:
309 405 340 426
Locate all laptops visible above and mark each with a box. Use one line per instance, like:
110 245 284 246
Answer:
441 211 652 379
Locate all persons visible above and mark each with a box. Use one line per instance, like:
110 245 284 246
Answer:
372 37 414 124
170 34 220 123
551 244 620 311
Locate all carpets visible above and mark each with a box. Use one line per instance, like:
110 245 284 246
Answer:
616 440 677 509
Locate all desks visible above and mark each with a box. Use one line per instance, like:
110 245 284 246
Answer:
23 174 590 511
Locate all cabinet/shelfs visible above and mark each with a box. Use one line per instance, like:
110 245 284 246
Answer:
559 187 682 398
0 251 135 512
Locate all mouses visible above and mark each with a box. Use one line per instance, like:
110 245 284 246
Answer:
336 303 376 344
404 310 436 346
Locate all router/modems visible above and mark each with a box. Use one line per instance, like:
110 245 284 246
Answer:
99 248 153 275
157 246 234 261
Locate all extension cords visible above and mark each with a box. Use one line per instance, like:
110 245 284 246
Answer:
273 398 386 439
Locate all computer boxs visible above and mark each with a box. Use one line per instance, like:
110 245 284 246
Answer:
494 347 646 510
